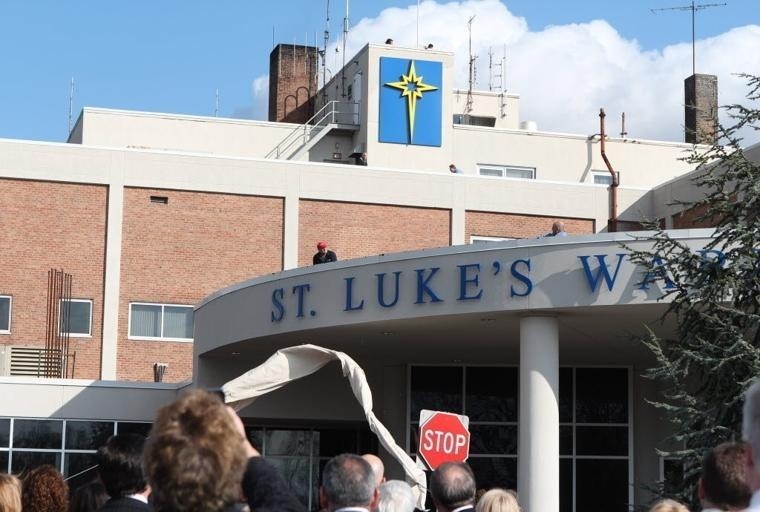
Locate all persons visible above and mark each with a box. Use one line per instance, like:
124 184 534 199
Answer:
423 43 434 49
449 164 462 173
698 442 760 511
357 151 366 164
313 242 337 265
537 221 566 240
384 38 394 44
649 497 690 512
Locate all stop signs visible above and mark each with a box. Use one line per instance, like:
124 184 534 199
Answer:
419 412 473 476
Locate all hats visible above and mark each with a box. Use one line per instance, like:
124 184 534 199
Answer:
317 241 328 251
